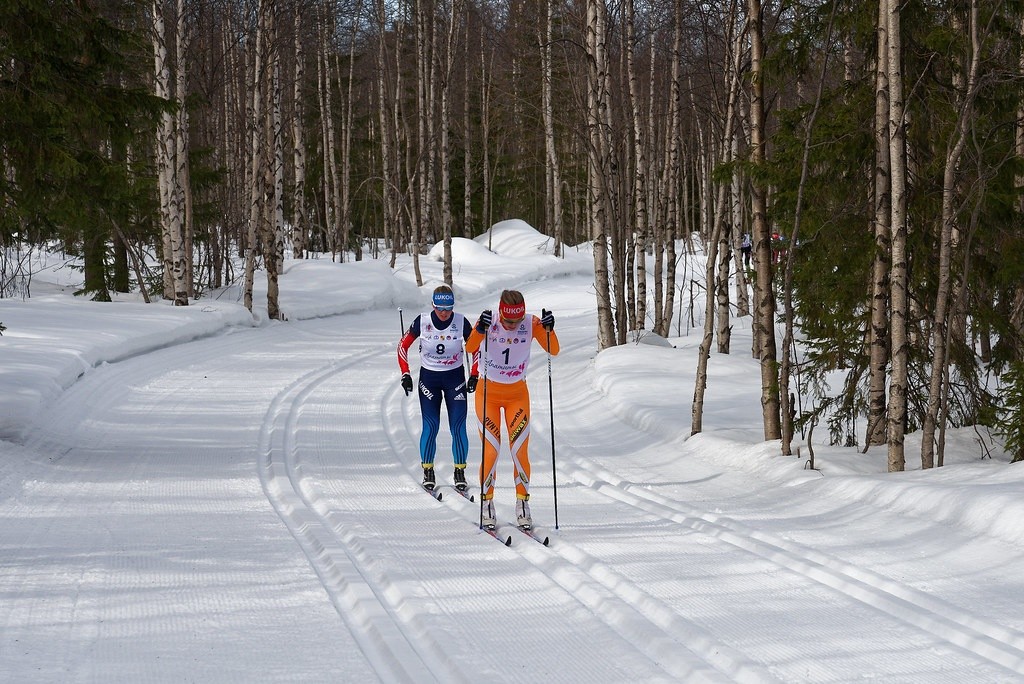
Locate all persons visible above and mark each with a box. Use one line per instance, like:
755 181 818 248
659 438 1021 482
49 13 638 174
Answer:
396 283 479 490
465 289 558 532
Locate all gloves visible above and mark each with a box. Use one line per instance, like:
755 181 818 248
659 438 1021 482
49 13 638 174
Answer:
476 309 493 334
467 376 478 393
401 374 412 396
541 308 555 332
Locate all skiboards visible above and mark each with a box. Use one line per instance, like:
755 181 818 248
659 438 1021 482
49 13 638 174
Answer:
416 481 474 503
471 520 550 546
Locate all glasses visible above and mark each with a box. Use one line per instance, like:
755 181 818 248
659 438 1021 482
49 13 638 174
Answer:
500 309 525 324
432 300 455 311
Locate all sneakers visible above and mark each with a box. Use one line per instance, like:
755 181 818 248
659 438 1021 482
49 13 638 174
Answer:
423 467 435 490
516 500 531 528
454 468 467 491
482 500 495 529
